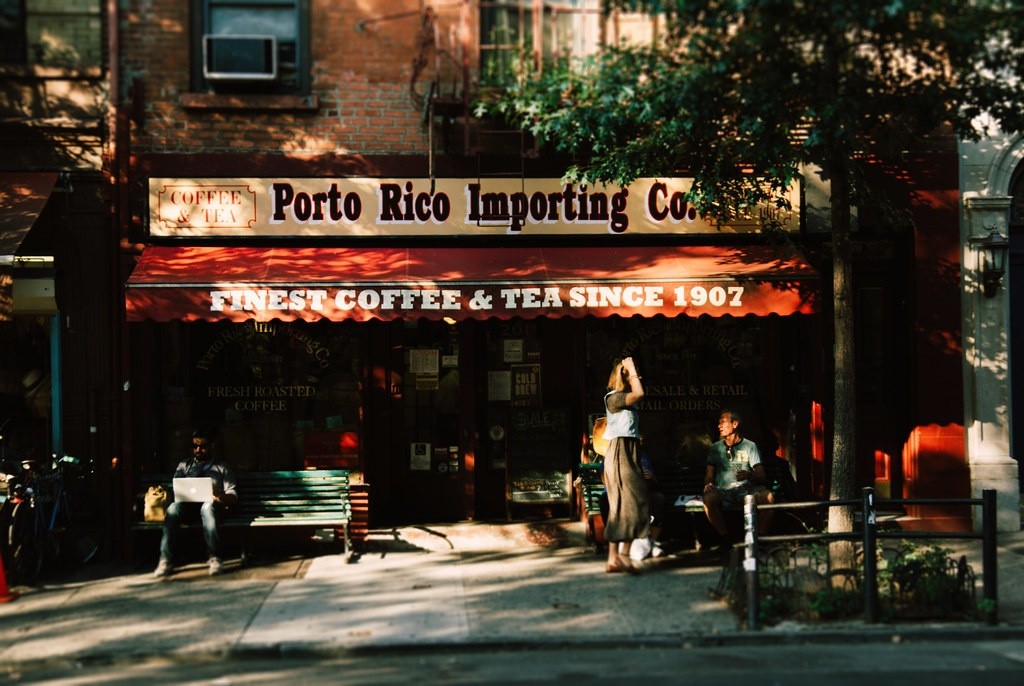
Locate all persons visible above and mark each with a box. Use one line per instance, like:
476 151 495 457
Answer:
150 429 238 579
701 408 775 556
592 356 661 573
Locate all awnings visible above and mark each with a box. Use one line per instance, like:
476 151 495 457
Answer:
123 240 823 322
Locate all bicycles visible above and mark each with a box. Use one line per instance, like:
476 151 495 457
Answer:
0 449 108 584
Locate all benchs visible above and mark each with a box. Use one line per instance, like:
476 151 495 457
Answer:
129 470 354 563
578 459 786 551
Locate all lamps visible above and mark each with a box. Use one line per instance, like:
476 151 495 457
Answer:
978 223 1009 299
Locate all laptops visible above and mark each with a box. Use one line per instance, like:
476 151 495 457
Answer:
173 476 213 503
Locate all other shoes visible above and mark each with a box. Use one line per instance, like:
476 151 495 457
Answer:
153 560 174 578
606 553 638 576
206 556 223 576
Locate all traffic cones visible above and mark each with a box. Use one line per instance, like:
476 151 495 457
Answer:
0 549 20 603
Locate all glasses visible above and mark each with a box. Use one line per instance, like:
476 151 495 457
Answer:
726 446 733 462
192 443 211 449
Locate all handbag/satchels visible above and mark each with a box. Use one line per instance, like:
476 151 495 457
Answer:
144 486 168 522
592 417 608 457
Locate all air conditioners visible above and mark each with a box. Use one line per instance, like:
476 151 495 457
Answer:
202 33 278 81
